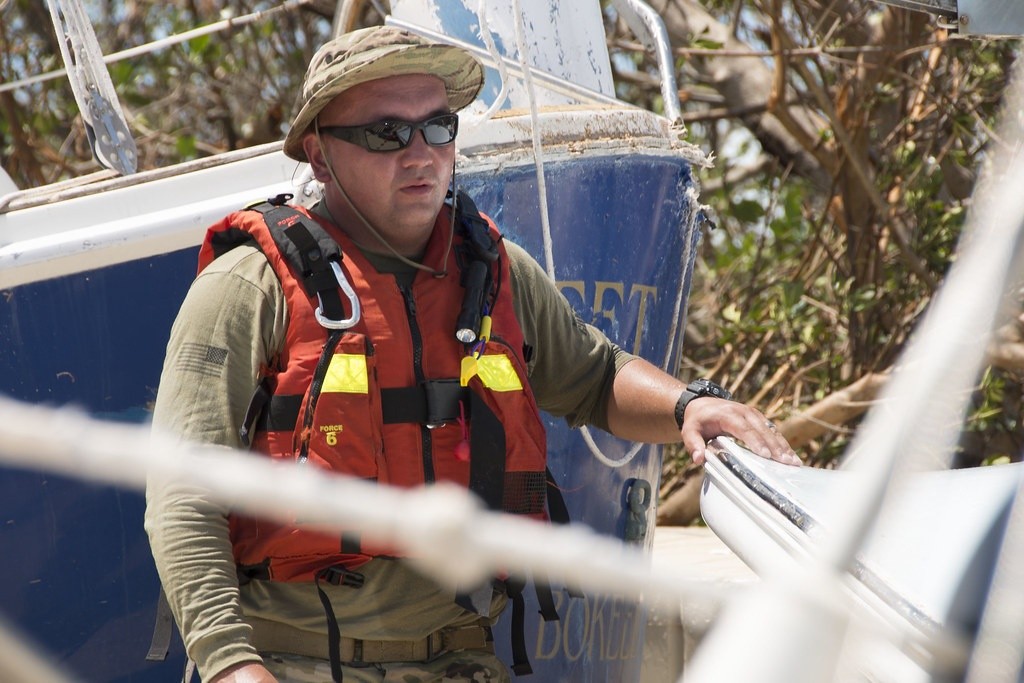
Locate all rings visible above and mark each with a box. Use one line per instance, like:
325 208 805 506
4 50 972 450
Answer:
764 419 777 431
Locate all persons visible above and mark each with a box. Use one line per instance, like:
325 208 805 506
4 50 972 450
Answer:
144 23 804 683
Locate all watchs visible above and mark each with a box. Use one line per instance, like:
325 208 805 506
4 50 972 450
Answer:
674 377 732 431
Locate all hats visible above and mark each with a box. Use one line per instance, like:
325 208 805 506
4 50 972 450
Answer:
283 24 488 163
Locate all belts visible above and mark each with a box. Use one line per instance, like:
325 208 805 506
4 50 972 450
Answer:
180 614 495 683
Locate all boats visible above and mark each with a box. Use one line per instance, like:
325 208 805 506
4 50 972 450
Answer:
0 1 719 683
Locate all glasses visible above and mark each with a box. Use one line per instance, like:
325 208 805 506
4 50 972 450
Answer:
318 114 459 154
